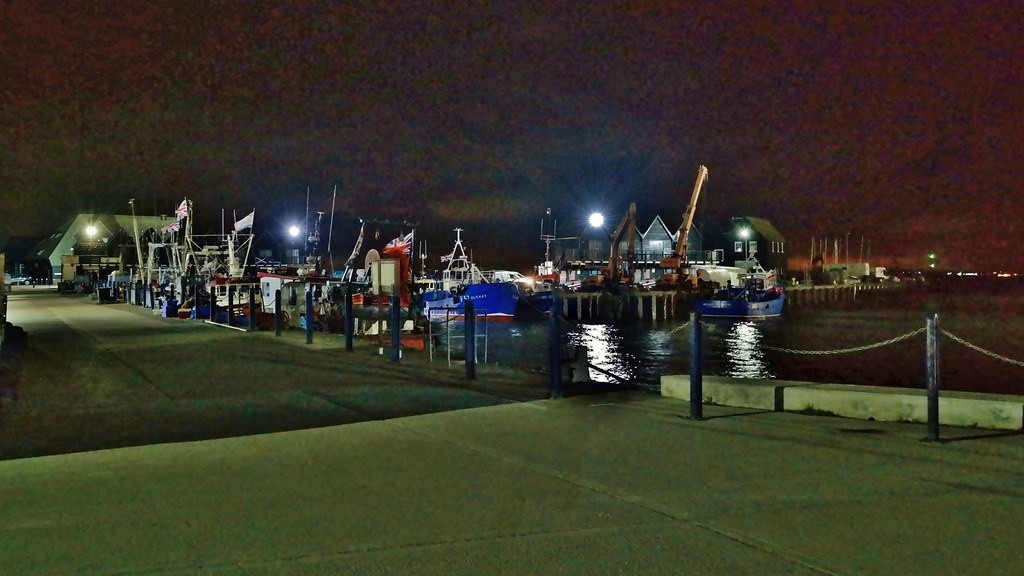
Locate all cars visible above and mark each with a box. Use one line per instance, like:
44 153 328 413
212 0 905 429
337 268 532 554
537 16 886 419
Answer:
9 274 34 286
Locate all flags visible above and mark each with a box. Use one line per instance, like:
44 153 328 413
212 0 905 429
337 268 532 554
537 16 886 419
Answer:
383 233 412 255
344 226 364 266
441 254 452 262
234 212 254 231
766 269 776 280
200 261 215 274
161 222 179 234
175 200 187 221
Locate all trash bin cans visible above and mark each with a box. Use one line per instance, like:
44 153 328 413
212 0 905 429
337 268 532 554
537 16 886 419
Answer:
95 287 111 304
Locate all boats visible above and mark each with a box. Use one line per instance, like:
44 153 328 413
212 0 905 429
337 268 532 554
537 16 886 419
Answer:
115 183 786 352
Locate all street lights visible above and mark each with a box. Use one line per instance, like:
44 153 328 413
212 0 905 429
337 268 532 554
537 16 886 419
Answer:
86 226 97 267
740 228 749 262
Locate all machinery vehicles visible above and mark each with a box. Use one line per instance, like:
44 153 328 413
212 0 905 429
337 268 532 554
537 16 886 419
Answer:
577 202 640 292
653 164 711 288
